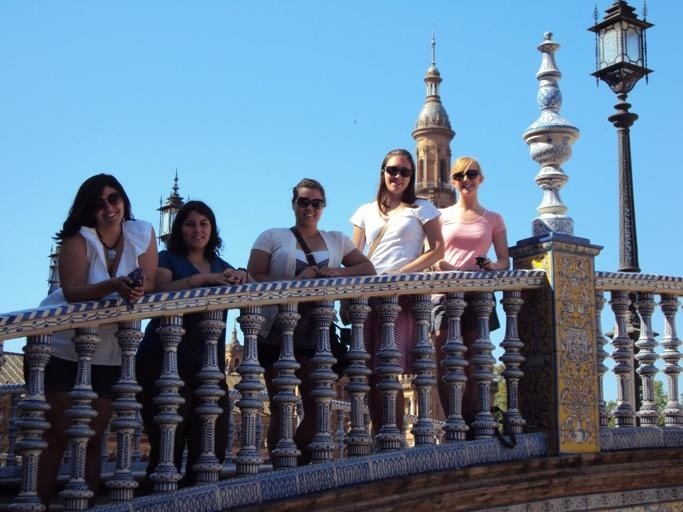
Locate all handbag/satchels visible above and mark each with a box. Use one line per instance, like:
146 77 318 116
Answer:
468 409 516 448
293 227 347 373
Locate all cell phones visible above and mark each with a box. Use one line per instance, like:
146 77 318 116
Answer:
475 256 486 269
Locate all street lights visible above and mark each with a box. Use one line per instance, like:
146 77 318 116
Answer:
588 0 655 426
155 167 187 246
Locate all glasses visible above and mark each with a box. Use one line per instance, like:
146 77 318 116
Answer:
93 192 122 209
294 197 323 210
452 172 482 182
384 166 412 178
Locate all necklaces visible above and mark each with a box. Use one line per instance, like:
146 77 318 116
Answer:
95 226 124 261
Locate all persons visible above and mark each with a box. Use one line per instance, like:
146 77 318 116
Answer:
25 174 158 504
247 179 376 457
136 201 252 490
348 150 445 428
426 158 510 417
